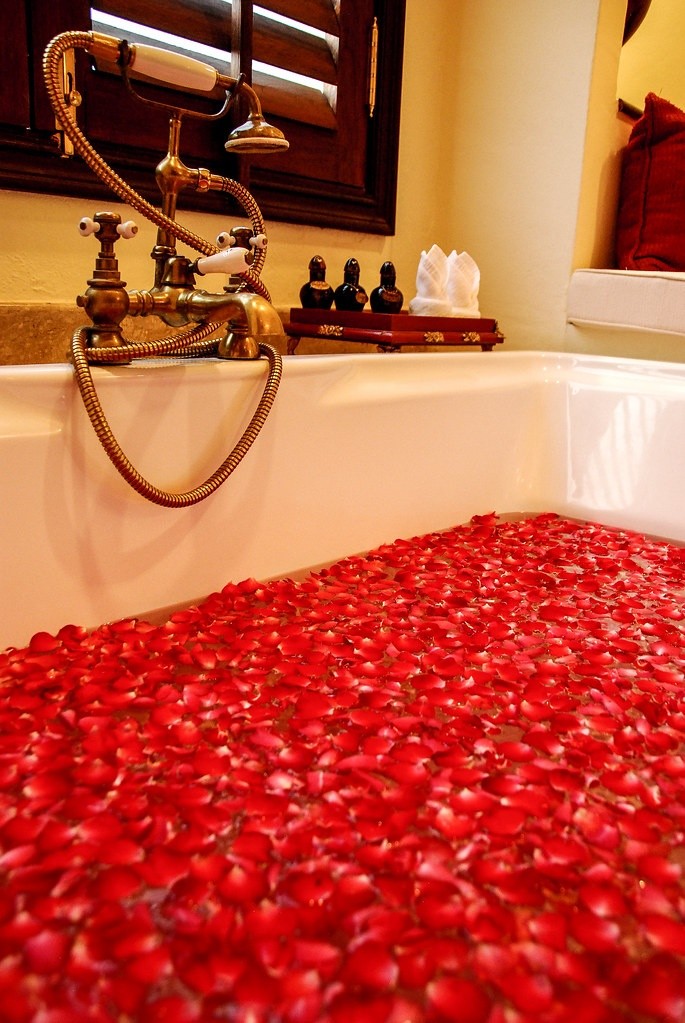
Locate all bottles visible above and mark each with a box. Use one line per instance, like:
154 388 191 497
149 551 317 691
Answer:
300 255 334 310
334 258 368 313
369 261 404 315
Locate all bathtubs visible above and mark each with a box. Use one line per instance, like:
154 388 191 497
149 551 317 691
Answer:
1 347 685 1021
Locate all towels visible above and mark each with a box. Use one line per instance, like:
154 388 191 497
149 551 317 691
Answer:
409 242 483 318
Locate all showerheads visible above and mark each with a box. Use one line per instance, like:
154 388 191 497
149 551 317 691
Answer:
87 27 291 156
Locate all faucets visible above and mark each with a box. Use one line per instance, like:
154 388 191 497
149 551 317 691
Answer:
158 247 287 342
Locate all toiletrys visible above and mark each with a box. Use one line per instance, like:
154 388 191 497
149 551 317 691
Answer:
298 254 404 314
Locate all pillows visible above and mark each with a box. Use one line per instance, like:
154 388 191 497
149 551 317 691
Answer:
617 88 685 273
